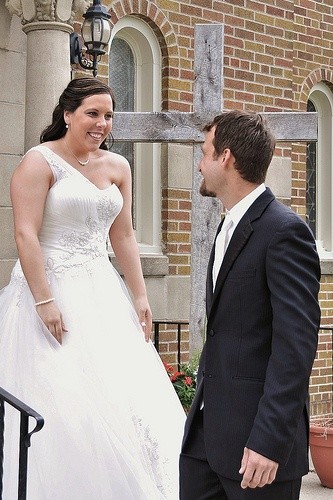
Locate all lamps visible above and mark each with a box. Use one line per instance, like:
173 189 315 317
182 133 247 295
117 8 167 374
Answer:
70 0 112 78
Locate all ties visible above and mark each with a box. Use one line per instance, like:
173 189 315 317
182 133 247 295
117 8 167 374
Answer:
212 214 233 292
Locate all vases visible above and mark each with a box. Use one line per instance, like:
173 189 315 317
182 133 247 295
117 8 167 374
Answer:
309 418 333 487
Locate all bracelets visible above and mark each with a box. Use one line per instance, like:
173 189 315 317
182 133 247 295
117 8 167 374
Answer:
34 298 54 306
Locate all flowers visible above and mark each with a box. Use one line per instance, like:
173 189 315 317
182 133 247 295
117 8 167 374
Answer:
160 349 202 417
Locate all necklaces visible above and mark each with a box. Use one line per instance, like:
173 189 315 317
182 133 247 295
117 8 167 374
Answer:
70 148 90 166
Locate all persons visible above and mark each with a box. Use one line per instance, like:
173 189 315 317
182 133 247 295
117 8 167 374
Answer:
0 77 188 500
178 109 321 500
